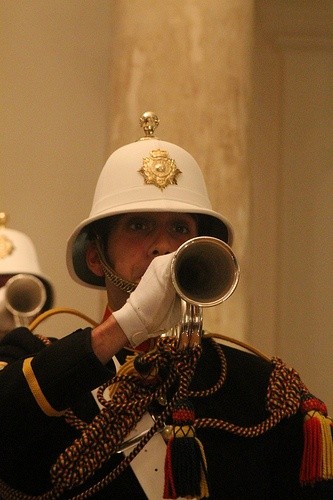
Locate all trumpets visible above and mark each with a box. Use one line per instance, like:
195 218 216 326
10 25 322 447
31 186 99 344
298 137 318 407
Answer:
2 273 47 327
160 234 242 359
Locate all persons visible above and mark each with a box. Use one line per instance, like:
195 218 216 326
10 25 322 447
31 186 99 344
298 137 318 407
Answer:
1 110 333 500
1 212 53 360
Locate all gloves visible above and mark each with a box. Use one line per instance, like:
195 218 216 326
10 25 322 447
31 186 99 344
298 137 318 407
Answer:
109 252 189 349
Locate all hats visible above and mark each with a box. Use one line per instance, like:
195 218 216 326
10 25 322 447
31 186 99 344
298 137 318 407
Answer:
65 111 233 288
0 210 55 315
